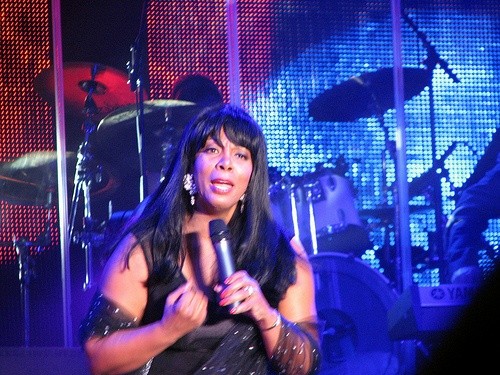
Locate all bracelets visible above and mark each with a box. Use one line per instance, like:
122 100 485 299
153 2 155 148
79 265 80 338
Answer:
260 313 281 332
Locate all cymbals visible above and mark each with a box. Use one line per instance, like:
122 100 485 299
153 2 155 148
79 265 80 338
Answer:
93 98 204 179
355 204 441 220
33 61 149 124
0 150 122 209
307 67 434 122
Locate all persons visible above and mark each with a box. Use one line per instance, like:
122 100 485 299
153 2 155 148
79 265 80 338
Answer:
447 151 500 283
167 75 221 127
77 102 322 375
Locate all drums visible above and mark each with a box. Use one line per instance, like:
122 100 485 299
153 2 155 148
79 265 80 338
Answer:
266 166 372 258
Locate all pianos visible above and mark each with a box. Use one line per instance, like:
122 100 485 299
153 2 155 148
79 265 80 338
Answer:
384 281 480 342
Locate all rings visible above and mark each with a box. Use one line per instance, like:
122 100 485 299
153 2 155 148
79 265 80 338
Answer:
244 286 253 296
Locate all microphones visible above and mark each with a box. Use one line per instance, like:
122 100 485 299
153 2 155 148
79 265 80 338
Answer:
440 142 456 162
209 218 240 308
